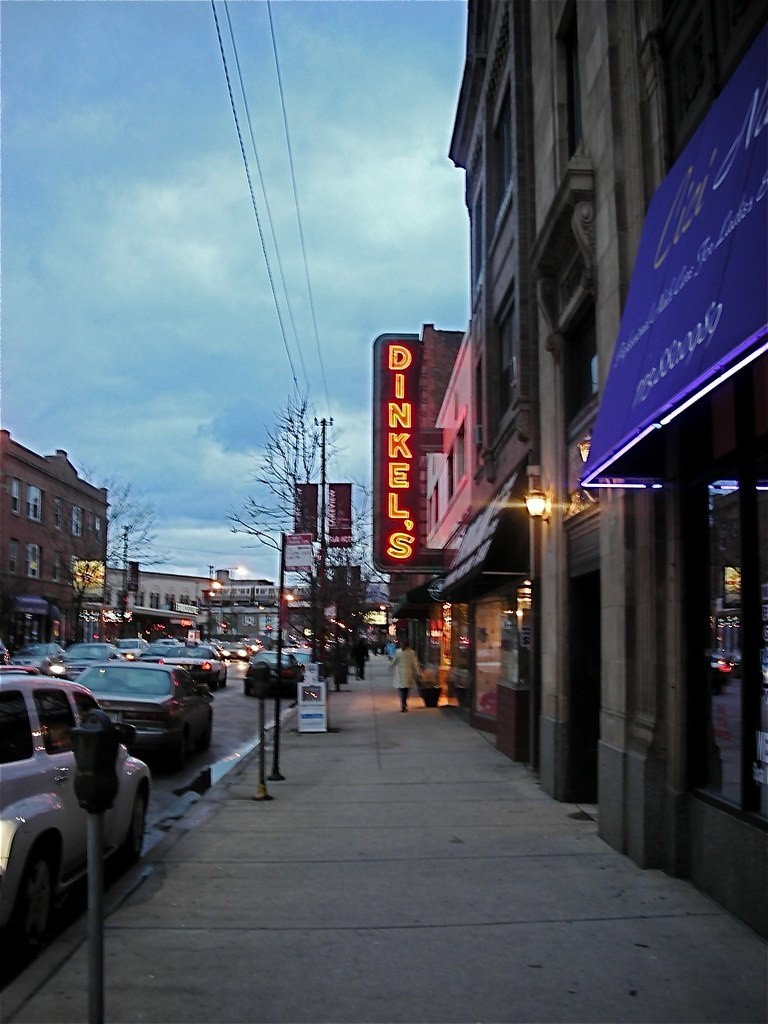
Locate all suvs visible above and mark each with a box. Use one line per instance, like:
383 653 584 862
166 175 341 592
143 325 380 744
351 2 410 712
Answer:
0 665 152 959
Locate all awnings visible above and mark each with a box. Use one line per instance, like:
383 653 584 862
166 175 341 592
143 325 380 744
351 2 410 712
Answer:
580 17 768 486
435 466 524 593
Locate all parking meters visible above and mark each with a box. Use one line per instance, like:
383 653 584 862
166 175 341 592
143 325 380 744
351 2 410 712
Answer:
252 661 274 800
74 710 126 1021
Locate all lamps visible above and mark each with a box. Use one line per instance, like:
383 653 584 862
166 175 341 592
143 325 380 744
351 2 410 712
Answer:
577 428 592 461
524 489 550 526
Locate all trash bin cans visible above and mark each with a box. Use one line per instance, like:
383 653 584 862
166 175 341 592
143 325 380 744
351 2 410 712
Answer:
305 662 325 682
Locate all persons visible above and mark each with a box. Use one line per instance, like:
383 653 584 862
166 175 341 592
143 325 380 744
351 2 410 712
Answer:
351 635 420 713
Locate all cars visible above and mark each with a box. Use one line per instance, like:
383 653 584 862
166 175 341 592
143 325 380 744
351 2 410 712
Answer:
116 638 151 663
243 650 308 696
287 651 314 672
8 642 66 676
138 644 180 663
154 635 273 660
0 639 11 665
72 662 215 773
46 642 131 684
159 643 228 690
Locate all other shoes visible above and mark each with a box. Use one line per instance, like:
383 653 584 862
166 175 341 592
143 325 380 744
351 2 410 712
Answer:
401 705 408 712
